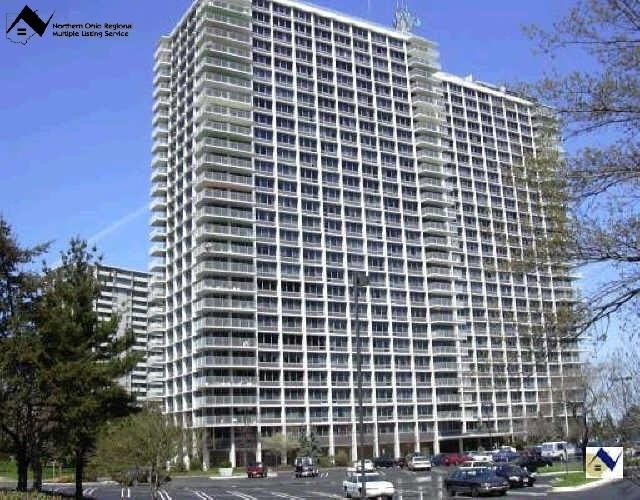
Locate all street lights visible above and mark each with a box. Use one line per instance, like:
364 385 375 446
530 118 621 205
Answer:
354 270 369 494
608 376 632 409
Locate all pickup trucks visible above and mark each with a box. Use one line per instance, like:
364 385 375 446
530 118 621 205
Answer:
112 465 171 486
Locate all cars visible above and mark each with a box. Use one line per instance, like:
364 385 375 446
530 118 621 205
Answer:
296 457 320 478
247 462 268 478
624 440 640 457
576 440 603 456
342 440 575 499
